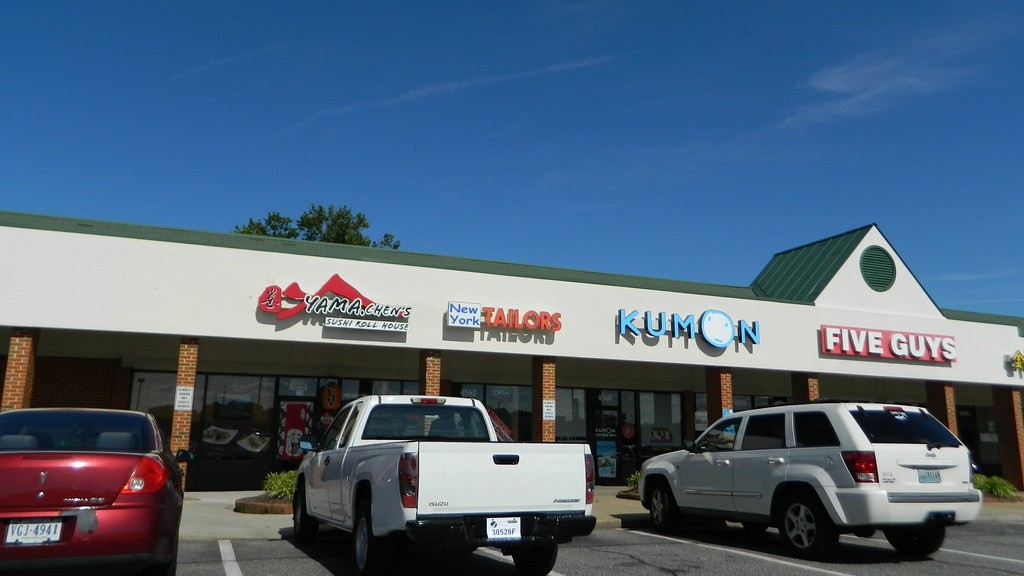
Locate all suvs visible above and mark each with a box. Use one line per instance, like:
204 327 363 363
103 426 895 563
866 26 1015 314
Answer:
637 402 983 556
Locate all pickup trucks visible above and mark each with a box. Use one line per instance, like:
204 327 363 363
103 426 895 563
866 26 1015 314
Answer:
292 394 598 576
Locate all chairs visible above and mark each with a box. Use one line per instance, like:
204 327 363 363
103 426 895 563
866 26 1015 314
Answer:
94 432 140 451
429 419 459 437
0 435 37 450
366 416 418 437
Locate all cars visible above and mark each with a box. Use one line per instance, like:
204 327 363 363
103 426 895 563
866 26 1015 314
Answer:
0 408 195 576
